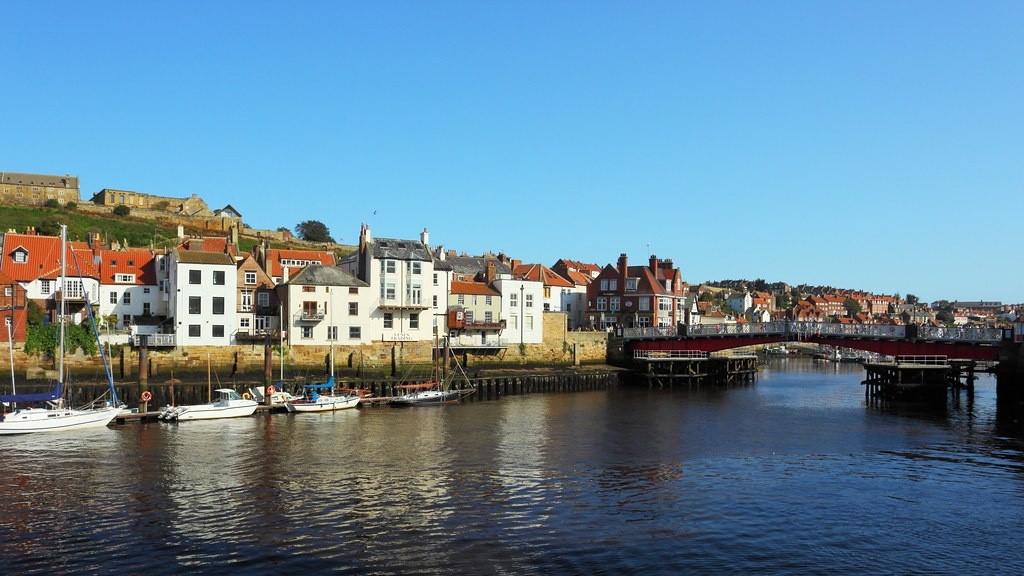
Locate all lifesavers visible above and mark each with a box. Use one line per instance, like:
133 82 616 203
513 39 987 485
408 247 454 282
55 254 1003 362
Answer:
267 386 276 396
141 391 152 402
242 392 250 400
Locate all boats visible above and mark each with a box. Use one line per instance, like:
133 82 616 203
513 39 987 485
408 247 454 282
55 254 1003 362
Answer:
385 326 477 408
156 354 306 421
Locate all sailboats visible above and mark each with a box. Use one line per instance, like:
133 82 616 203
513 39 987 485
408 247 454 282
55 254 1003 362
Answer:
283 289 361 413
0 225 127 436
